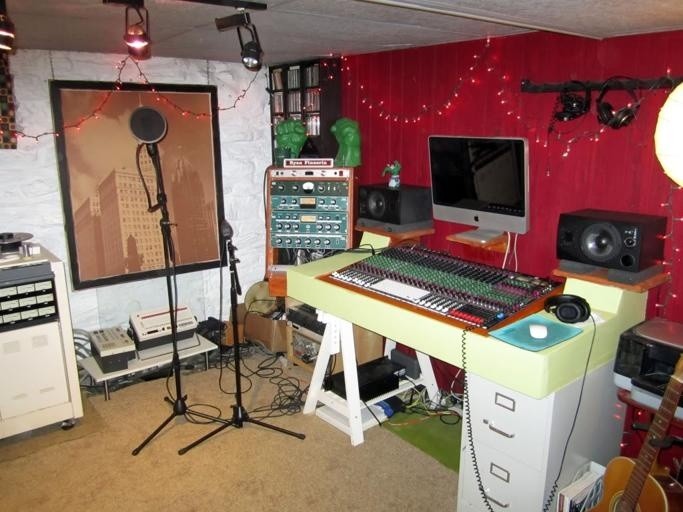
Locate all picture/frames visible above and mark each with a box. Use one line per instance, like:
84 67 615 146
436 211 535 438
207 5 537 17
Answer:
48 79 228 292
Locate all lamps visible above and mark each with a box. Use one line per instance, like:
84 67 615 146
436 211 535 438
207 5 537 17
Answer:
215 12 264 72
122 5 153 60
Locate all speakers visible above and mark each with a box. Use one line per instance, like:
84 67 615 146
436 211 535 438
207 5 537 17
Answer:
357 182 431 234
556 207 668 273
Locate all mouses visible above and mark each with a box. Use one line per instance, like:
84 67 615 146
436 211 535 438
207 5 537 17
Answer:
528 322 547 339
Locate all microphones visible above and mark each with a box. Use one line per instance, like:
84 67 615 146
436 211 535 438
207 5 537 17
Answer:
130 106 168 158
221 221 233 255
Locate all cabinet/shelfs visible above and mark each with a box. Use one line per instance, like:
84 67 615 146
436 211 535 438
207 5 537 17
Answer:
457 362 614 511
285 297 384 376
0 320 69 419
267 55 340 159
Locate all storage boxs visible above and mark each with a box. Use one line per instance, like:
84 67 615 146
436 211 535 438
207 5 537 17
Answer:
230 304 287 352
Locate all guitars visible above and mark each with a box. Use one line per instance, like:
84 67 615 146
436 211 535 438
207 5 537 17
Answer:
584 349 683 512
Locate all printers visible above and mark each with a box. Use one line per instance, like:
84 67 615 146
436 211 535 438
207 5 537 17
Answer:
610 317 683 420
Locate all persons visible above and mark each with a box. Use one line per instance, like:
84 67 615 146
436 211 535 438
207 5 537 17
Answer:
275 118 308 158
330 117 363 167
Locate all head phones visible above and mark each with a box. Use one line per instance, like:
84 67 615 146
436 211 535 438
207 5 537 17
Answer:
556 80 591 121
545 295 592 324
598 77 639 127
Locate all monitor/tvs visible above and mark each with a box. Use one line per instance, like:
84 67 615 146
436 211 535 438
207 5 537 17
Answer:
428 134 530 244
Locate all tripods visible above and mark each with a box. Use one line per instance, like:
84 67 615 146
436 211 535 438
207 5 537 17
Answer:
127 194 239 458
179 252 306 456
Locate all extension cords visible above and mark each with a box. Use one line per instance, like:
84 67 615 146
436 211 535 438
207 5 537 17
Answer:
554 464 609 512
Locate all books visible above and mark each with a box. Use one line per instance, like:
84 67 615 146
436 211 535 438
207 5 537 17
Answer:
283 158 333 170
271 91 284 113
287 65 301 89
305 113 320 136
304 89 320 112
290 113 301 121
271 68 284 90
272 115 284 135
287 91 302 112
304 63 320 88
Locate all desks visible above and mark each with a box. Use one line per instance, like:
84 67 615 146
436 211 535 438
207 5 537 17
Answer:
78 331 218 401
